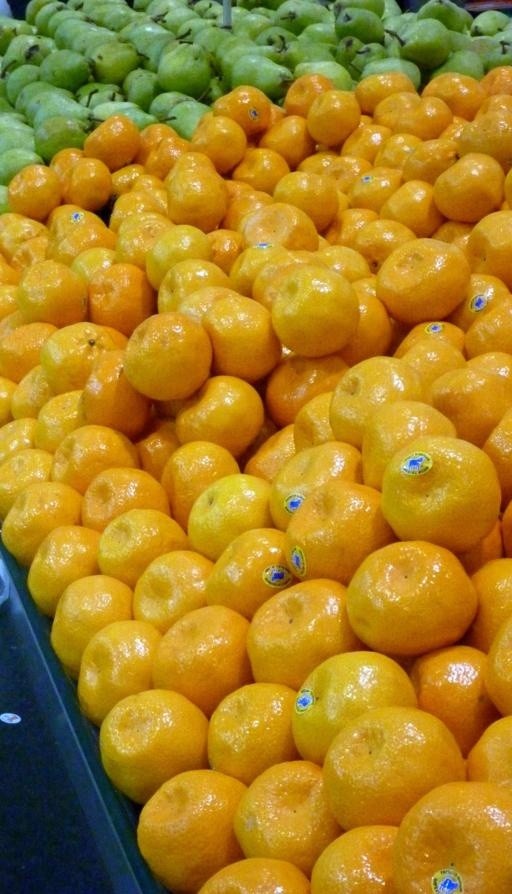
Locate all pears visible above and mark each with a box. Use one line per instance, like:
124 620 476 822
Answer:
0 0 512 211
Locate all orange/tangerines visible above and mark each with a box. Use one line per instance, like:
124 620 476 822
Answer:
0 66 512 894
